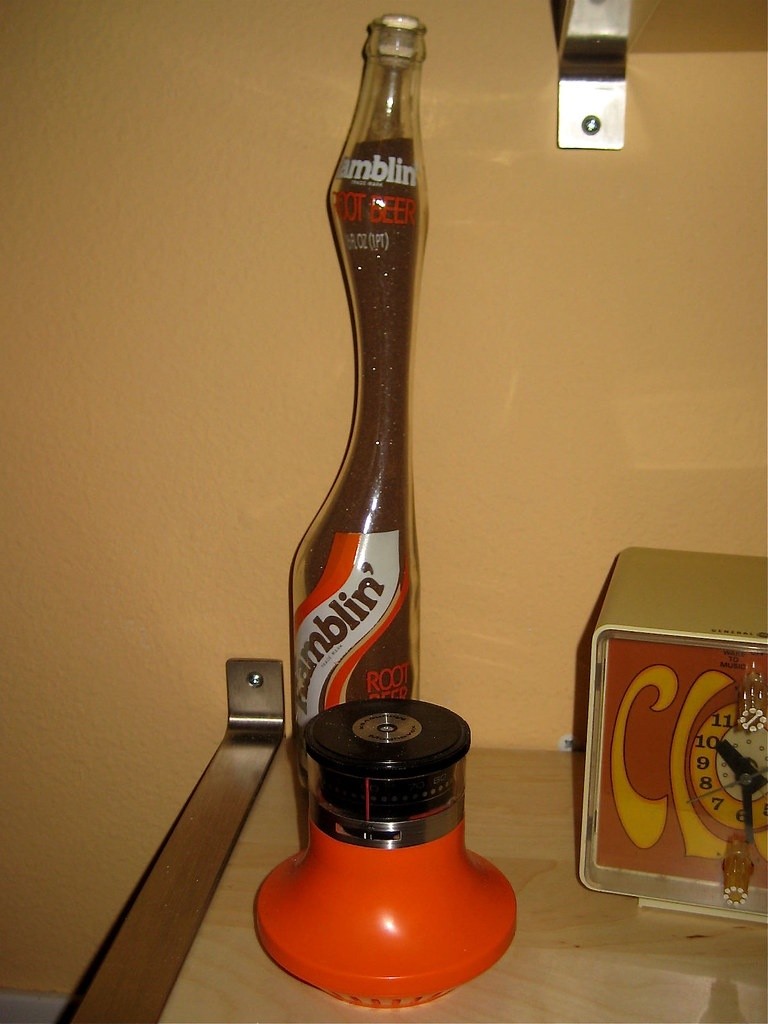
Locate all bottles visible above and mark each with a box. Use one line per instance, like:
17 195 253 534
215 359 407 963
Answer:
284 14 427 795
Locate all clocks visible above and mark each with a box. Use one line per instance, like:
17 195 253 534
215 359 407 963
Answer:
579 547 768 927
